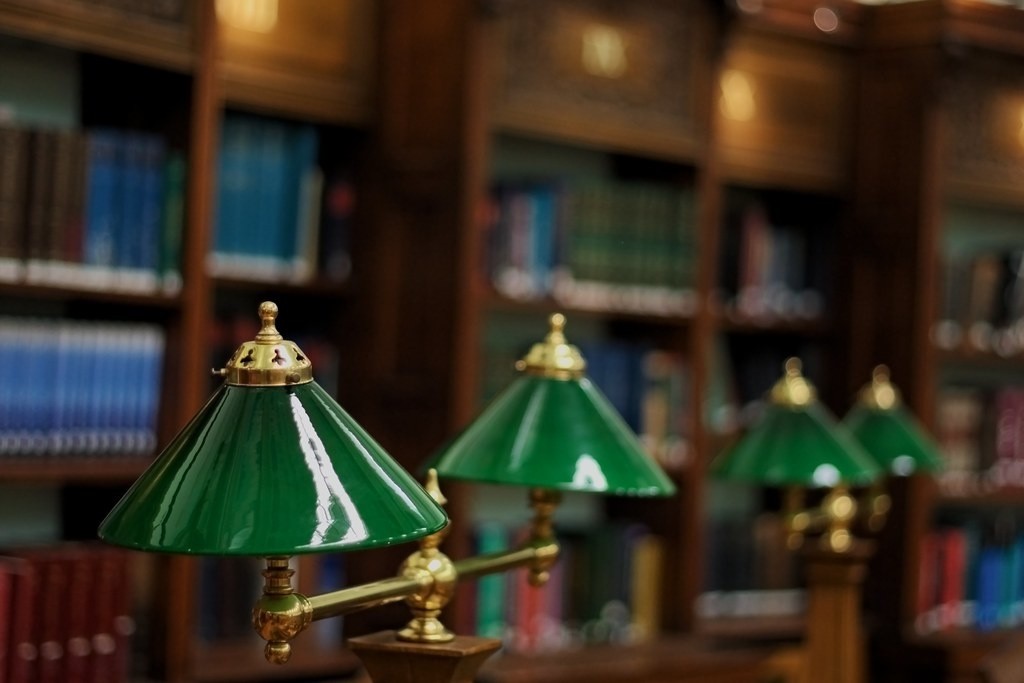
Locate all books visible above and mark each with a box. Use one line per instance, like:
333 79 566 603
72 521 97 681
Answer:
495 0 1024 198
0 122 186 297
207 320 344 646
0 543 156 683
699 191 1024 632
0 0 360 102
475 517 671 652
0 321 168 456
208 121 364 288
489 162 697 316
482 323 689 467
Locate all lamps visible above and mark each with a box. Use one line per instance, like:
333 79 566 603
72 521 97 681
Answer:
93 272 686 683
710 354 949 683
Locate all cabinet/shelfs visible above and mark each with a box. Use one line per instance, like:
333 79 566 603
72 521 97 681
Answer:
0 0 1024 683
